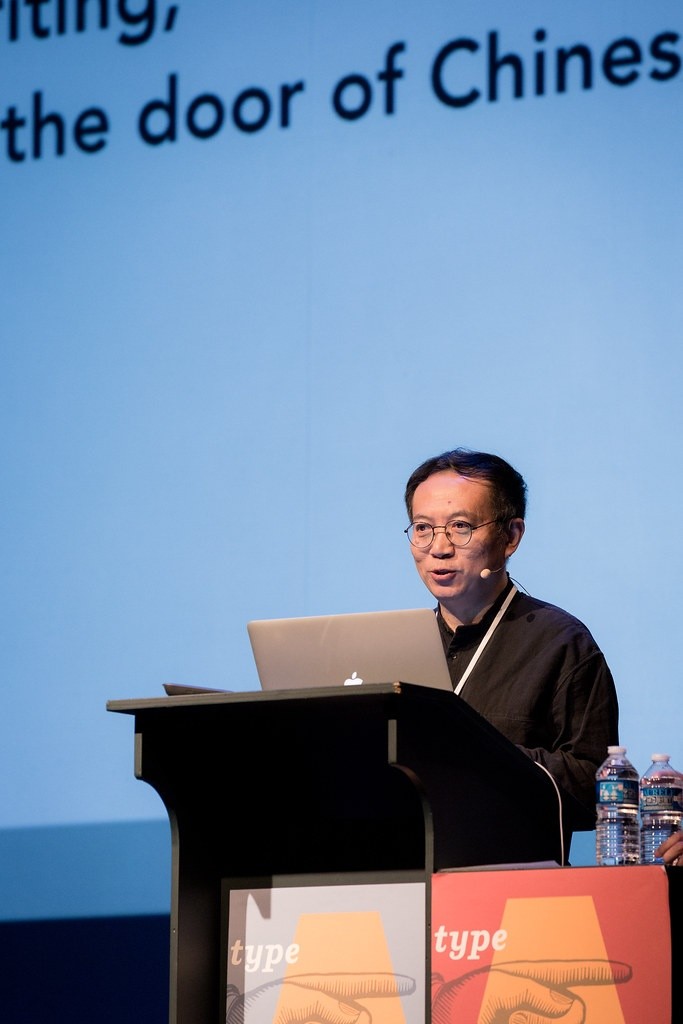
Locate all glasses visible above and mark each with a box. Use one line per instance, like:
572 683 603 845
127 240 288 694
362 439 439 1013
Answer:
404 519 498 549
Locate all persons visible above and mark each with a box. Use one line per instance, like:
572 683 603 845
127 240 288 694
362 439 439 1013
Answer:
404 449 620 869
655 831 683 866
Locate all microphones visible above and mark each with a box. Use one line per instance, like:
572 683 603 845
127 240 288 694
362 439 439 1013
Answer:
481 564 506 578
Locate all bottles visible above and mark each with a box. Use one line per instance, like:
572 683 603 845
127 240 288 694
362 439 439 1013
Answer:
639 754 683 864
597 745 641 865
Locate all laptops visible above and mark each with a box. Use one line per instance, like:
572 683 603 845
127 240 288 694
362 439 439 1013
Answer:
248 607 453 692
